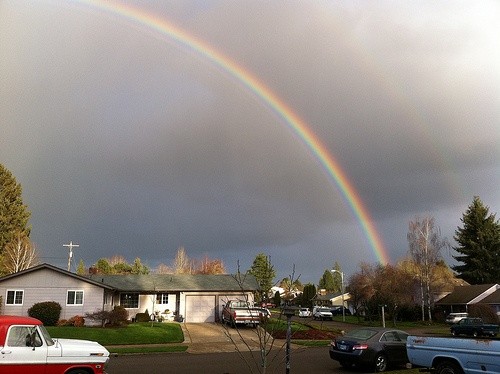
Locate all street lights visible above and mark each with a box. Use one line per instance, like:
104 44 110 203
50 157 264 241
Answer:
279 306 302 374
330 269 345 323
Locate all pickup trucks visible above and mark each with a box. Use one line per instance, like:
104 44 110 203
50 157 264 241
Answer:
448 316 500 336
221 300 268 328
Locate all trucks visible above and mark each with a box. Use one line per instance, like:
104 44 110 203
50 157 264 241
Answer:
406 334 500 373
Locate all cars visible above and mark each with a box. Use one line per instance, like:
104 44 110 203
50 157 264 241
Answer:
330 306 351 316
298 307 313 318
328 327 414 373
0 315 111 374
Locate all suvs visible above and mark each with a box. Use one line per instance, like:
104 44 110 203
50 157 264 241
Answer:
313 305 333 321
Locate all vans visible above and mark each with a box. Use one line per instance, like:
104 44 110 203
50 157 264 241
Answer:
446 313 468 325
